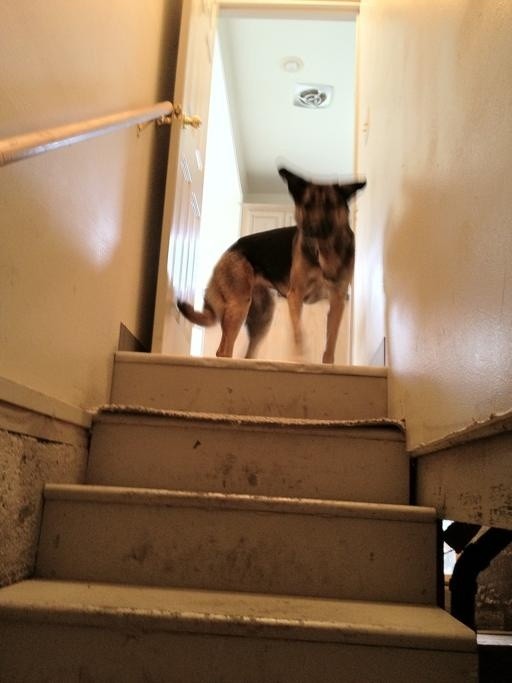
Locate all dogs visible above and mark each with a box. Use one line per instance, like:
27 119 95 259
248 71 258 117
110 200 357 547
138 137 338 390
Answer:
175 165 368 364
